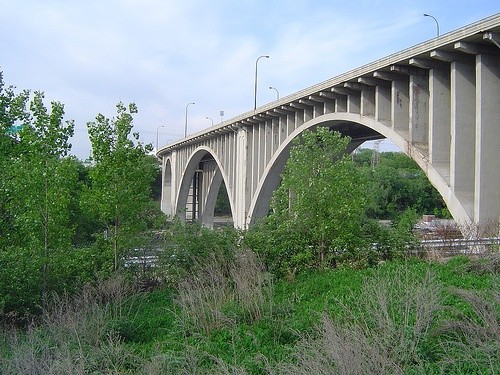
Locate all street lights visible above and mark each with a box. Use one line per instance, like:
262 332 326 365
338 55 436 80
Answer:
423 13 439 37
206 117 213 126
166 139 172 145
185 103 195 137
270 86 279 100
254 56 269 110
156 126 164 151
